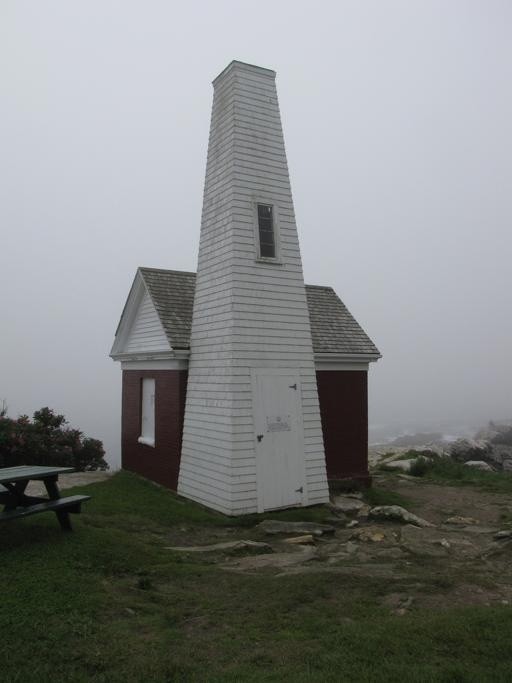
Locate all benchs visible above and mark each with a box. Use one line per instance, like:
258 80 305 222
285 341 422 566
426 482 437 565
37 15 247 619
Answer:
0 494 91 520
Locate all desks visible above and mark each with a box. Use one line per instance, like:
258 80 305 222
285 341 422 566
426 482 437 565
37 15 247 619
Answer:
0 465 75 532
